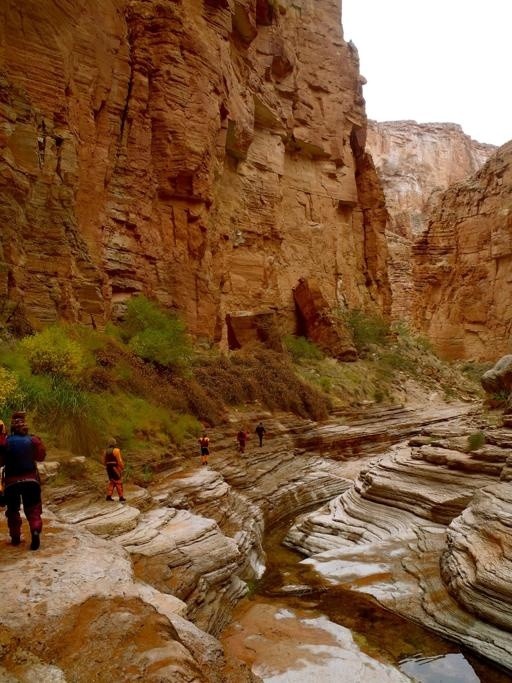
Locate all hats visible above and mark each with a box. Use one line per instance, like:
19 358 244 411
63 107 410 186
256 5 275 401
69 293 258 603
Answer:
11 417 26 433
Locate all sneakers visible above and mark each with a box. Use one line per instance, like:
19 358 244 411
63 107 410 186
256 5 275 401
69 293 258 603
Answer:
11 537 20 545
30 530 40 550
105 495 114 501
118 496 126 501
203 461 208 466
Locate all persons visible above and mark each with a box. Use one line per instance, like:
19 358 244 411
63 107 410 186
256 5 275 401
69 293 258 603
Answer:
1 410 47 551
255 422 267 447
101 437 128 502
236 427 250 454
197 432 211 466
